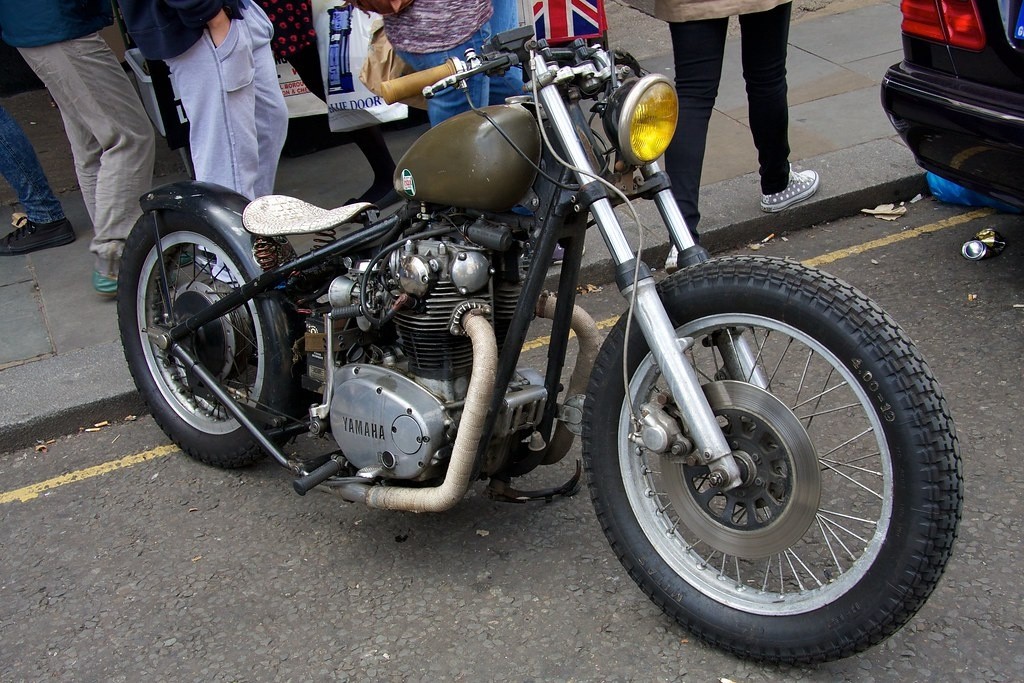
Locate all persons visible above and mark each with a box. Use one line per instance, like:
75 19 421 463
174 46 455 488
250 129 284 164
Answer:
1 1 396 293
352 0 586 266
655 0 819 275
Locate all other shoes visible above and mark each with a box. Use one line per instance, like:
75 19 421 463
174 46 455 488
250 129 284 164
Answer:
93 270 176 298
552 243 586 265
179 251 193 267
342 178 404 211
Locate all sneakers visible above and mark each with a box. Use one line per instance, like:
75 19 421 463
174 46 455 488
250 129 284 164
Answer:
665 244 679 275
760 163 820 213
0 217 77 256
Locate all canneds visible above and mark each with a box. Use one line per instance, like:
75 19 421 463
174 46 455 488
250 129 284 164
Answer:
961 227 1006 261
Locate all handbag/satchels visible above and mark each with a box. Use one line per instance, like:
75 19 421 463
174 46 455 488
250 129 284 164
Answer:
358 18 428 110
327 0 409 132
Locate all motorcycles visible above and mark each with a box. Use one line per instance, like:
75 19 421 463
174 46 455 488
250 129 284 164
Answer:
115 25 968 670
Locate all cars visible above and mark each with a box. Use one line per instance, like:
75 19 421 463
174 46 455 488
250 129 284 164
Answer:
879 0 1024 212
